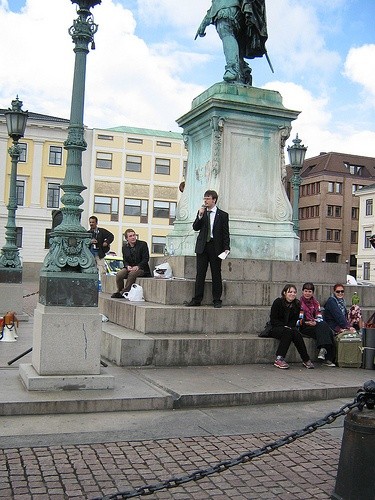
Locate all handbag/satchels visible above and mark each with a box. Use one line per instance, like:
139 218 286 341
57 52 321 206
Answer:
153 262 172 279
123 284 145 301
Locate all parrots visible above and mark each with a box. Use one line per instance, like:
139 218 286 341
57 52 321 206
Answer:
351 291 361 306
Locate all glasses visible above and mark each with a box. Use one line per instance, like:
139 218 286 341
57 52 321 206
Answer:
335 290 344 293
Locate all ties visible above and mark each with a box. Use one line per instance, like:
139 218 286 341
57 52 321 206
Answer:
207 211 212 242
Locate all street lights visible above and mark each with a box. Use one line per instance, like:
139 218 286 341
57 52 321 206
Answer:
286 133 308 237
0 94 30 283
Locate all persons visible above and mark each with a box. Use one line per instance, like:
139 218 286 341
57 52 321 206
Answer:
297 281 337 366
194 0 268 86
184 189 230 308
324 284 356 334
267 284 315 369
87 216 114 285
110 228 151 298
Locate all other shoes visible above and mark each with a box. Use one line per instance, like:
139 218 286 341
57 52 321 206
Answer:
214 302 222 308
109 292 124 298
273 359 290 369
187 300 202 306
304 362 315 368
324 360 336 367
318 348 326 360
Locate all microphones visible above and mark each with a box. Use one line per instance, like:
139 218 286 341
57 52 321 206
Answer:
200 204 205 217
